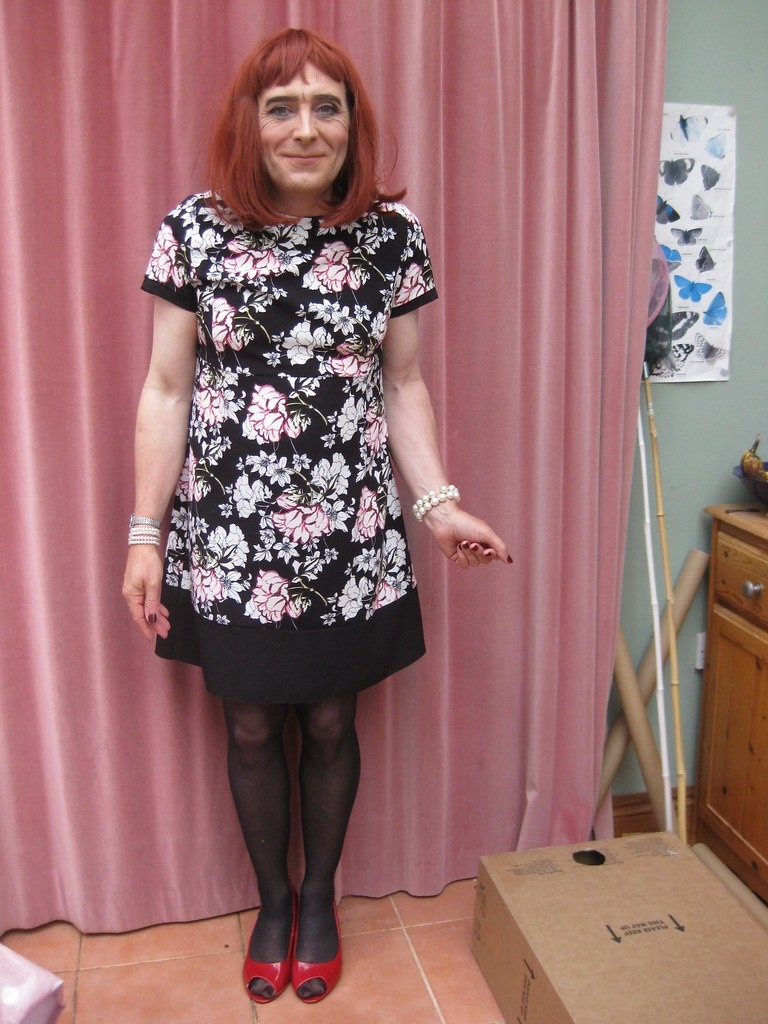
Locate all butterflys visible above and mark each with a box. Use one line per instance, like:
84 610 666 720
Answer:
646 113 731 377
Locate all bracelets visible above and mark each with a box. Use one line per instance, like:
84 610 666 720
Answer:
411 485 461 523
128 528 161 546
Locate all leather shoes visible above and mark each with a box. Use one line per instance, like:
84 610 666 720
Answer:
242 890 298 1004
291 890 341 1006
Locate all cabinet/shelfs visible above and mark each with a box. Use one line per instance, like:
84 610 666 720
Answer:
696 506 767 903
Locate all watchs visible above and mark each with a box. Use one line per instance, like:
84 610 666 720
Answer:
129 514 161 528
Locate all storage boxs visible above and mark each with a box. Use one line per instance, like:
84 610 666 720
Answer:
473 832 768 1024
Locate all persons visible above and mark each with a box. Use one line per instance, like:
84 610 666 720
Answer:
123 28 515 1004
